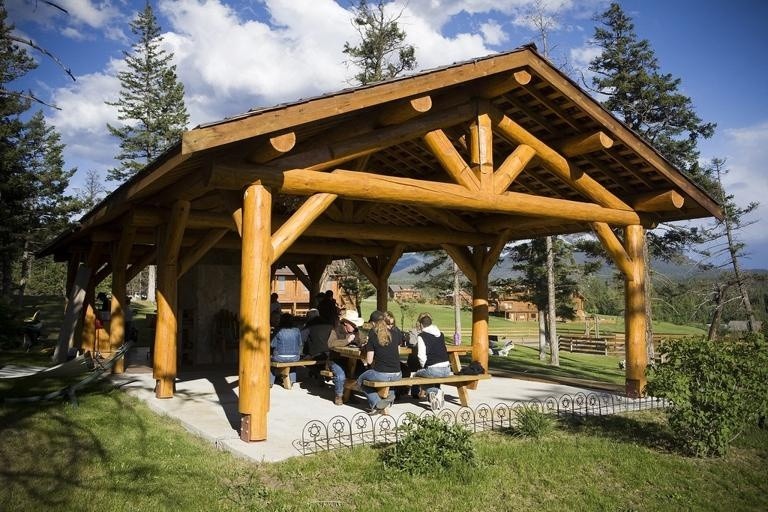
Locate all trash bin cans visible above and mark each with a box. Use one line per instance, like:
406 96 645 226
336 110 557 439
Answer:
488 335 497 348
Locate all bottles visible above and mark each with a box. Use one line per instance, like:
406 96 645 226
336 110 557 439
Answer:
454 331 459 347
400 331 411 347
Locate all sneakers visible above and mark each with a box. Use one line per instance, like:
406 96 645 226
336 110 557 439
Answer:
367 395 393 415
428 389 445 411
334 394 343 406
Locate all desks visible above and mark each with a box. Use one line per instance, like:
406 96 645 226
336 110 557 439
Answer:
329 343 474 413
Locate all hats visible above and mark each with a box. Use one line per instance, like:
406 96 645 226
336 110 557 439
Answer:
339 309 364 326
315 293 327 298
368 310 385 322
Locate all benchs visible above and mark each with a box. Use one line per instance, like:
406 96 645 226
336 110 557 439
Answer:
271 360 319 389
364 373 492 407
320 370 350 402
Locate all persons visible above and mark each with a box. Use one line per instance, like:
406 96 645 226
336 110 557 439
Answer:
94 291 111 311
356 309 404 416
125 295 133 345
326 309 369 406
410 315 451 412
299 307 334 379
270 310 282 326
407 312 434 346
314 291 337 324
325 289 334 306
270 292 281 311
381 310 406 346
268 311 304 388
